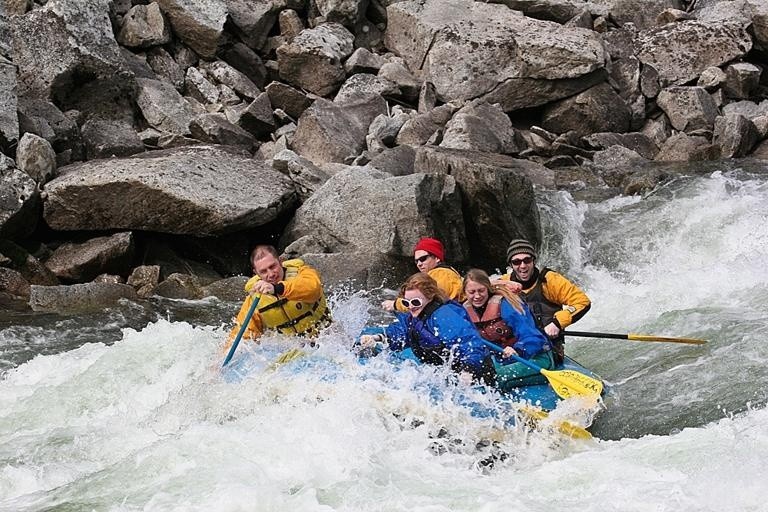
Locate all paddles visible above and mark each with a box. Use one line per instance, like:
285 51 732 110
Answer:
478 337 602 411
558 330 708 345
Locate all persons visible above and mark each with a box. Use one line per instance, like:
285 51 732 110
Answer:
380 237 468 314
463 269 556 393
488 235 594 367
359 272 499 398
220 244 335 375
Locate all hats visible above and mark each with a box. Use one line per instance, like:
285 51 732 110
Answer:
505 238 536 264
415 237 444 262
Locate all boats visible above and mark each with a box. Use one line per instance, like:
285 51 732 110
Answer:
218 327 609 466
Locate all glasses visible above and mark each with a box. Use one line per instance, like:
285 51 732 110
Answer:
400 297 424 309
510 255 532 265
414 254 431 264
